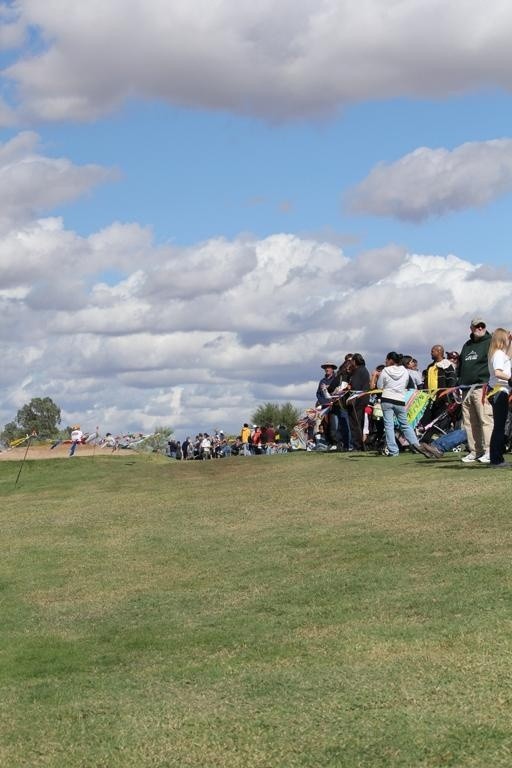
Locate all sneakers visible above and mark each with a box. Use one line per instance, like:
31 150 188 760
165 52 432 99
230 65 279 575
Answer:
460 451 491 463
412 442 444 459
491 462 509 468
166 446 399 460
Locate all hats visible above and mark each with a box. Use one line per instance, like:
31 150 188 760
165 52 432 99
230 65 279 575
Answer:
471 317 487 325
321 361 337 370
